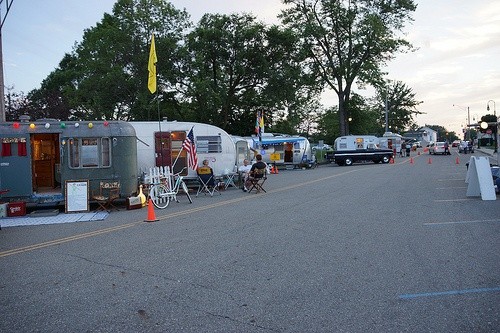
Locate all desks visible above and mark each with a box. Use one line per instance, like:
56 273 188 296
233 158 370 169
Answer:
221 172 239 190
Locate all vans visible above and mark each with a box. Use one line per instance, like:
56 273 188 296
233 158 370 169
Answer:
329 135 394 166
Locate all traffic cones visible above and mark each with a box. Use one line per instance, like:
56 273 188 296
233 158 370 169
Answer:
143 199 160 222
456 156 460 164
417 150 421 155
428 157 433 164
410 157 414 164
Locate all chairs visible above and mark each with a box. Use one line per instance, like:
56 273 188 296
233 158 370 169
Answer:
90 179 120 213
194 166 219 197
248 168 267 194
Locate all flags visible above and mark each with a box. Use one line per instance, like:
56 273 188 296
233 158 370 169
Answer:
147 34 158 94
255 112 264 135
182 127 198 170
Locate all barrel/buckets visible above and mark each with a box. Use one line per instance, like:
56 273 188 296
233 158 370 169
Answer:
0 203 7 218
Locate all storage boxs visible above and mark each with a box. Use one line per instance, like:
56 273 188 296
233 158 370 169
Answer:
8 201 26 216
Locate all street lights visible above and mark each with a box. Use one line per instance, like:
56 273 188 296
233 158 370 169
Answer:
487 100 498 154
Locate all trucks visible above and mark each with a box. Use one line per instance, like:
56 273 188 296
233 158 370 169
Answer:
0 115 237 207
231 133 313 169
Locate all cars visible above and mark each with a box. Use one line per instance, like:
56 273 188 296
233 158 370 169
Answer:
452 140 463 148
428 142 450 155
457 141 475 154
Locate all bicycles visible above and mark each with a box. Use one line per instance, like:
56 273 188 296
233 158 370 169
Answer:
150 167 193 209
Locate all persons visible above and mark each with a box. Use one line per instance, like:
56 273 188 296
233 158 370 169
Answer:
400 141 417 157
238 154 267 192
444 139 474 155
202 160 220 191
